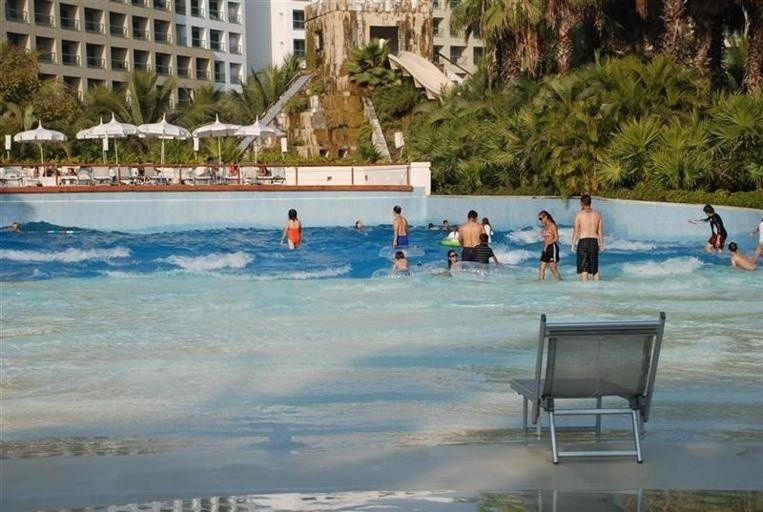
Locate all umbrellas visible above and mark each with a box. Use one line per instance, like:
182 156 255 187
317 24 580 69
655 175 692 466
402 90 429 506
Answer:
11 110 278 176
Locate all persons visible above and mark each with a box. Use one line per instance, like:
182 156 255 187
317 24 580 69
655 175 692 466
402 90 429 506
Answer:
354 219 363 229
7 222 21 233
745 216 763 263
728 241 757 271
687 203 728 253
537 209 562 281
280 208 302 250
392 251 410 277
391 204 409 252
32 157 272 186
571 194 605 282
427 209 500 277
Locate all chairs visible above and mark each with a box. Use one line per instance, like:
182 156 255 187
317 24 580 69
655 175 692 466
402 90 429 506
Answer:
0 165 287 188
509 312 666 463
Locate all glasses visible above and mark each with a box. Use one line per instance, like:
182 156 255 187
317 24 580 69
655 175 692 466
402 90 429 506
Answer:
450 254 458 257
539 217 544 221
